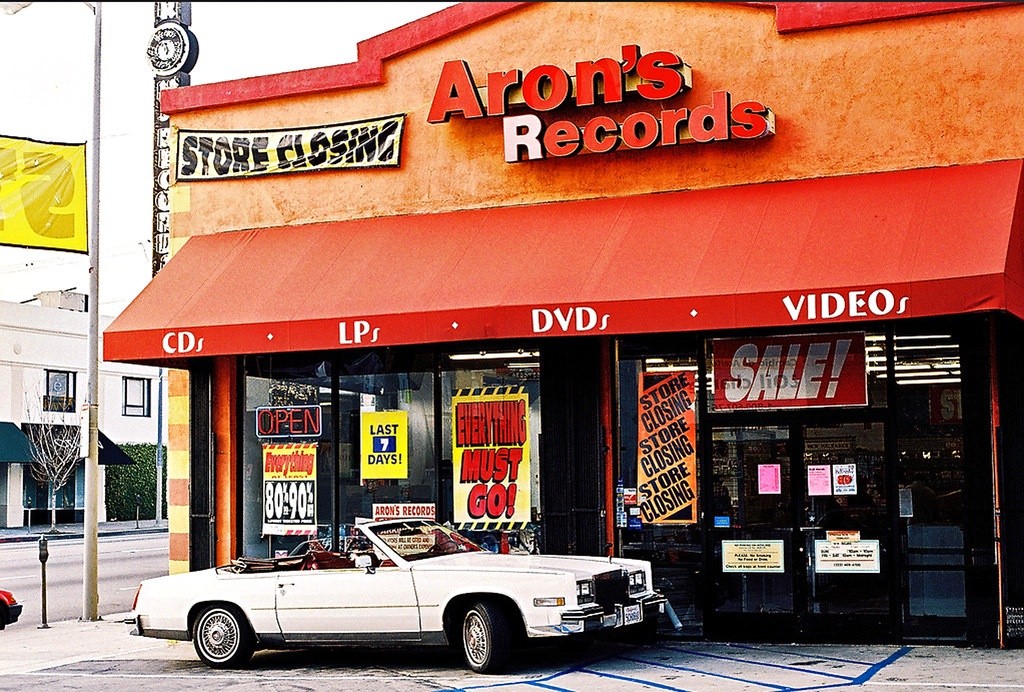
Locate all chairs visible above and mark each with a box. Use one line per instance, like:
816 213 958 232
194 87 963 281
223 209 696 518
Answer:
303 549 353 570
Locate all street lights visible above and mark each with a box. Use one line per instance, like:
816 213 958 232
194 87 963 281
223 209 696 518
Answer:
1 2 101 622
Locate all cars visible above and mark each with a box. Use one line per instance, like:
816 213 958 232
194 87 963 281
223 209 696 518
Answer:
129 519 667 674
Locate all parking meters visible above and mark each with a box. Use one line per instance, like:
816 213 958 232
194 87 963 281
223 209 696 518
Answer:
37 534 52 629
